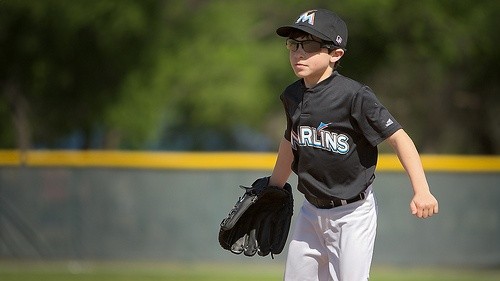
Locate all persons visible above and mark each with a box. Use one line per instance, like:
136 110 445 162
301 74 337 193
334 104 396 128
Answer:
268 8 439 281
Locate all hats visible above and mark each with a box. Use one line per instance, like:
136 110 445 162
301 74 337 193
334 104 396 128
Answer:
276 8 348 49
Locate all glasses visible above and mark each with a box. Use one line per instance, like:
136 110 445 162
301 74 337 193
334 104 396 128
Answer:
285 37 340 52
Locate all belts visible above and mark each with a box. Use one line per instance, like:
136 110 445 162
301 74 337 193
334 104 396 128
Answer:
304 193 365 208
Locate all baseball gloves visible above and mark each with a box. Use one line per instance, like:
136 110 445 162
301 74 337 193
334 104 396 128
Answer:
218 175 293 260
